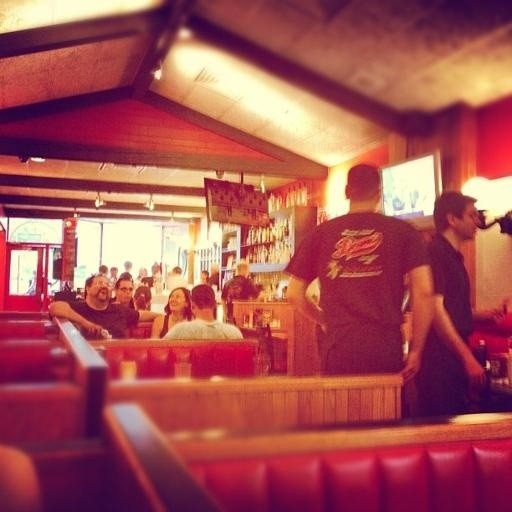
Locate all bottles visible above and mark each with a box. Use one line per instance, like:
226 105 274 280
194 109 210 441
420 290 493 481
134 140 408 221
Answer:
478 339 491 379
267 189 307 214
244 223 291 263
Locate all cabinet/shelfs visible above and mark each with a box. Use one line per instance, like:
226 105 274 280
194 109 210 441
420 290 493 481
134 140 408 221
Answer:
219 205 317 292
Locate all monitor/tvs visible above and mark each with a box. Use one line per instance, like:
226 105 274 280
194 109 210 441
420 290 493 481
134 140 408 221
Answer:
380 148 442 230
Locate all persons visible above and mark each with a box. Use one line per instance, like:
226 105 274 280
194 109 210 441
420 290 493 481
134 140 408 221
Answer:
412 189 487 424
26 261 268 342
281 161 435 425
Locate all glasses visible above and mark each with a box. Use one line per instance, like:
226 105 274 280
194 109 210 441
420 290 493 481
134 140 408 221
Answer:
118 286 135 293
95 280 115 289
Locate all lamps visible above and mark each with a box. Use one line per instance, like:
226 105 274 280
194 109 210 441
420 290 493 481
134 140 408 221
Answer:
95 192 107 209
143 193 155 212
